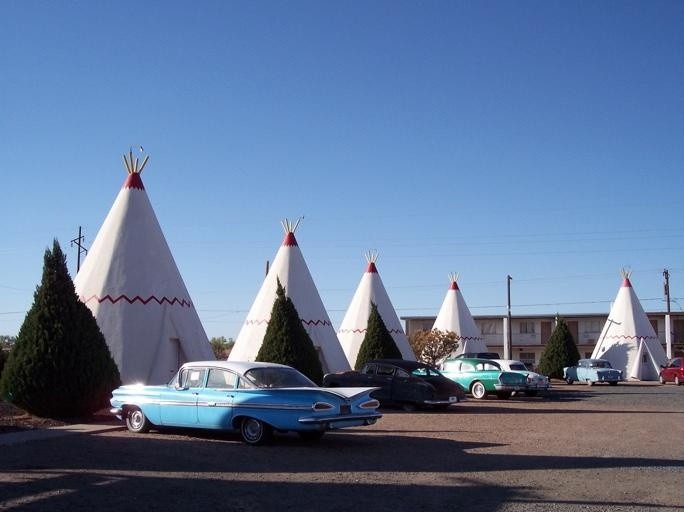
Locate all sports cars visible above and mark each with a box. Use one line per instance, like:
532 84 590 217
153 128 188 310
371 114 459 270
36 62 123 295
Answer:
321 358 465 414
107 361 382 446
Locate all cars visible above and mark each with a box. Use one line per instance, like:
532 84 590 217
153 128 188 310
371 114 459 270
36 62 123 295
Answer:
658 357 683 386
411 356 526 400
486 359 550 398
562 358 623 388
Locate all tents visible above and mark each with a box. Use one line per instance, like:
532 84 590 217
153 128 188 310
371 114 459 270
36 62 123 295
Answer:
589 270 669 381
73 152 489 388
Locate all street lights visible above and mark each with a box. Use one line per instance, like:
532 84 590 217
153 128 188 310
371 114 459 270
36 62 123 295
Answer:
506 275 512 358
